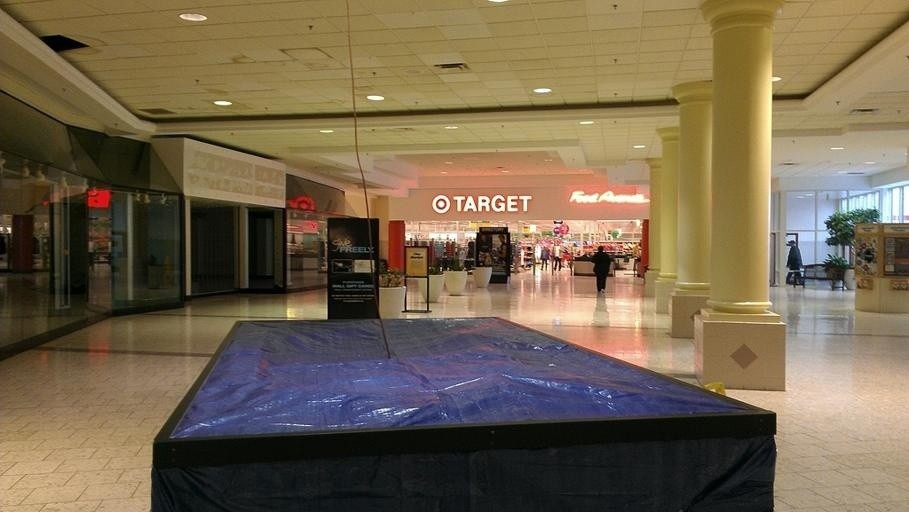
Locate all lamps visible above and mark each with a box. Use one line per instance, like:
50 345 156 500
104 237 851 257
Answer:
58 170 88 194
0 150 47 183
135 189 168 205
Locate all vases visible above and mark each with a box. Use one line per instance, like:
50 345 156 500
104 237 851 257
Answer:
471 267 493 288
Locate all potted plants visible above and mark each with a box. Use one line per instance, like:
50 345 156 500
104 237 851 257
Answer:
419 251 469 302
824 204 880 291
377 262 407 319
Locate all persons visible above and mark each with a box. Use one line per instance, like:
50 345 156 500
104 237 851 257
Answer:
539 246 549 271
786 240 804 286
552 242 563 270
478 235 491 266
492 233 507 264
590 245 611 293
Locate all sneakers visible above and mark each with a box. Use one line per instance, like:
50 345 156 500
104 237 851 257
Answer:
598 289 605 293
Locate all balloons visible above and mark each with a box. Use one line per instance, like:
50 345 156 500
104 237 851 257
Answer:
553 227 560 234
612 230 619 238
560 223 570 234
